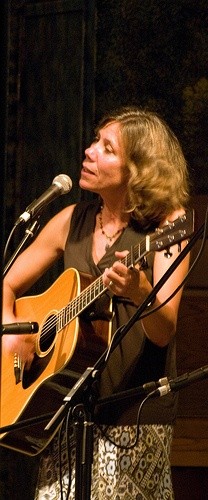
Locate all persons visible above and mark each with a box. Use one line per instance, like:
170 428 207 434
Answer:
0 106 192 500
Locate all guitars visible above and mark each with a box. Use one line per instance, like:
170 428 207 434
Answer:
0 206 197 458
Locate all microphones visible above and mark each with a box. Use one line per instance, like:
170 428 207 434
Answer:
2 321 39 334
147 367 208 398
14 173 73 227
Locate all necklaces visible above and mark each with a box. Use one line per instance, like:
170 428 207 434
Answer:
99 205 125 242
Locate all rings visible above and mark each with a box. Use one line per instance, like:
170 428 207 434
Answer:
107 280 113 287
